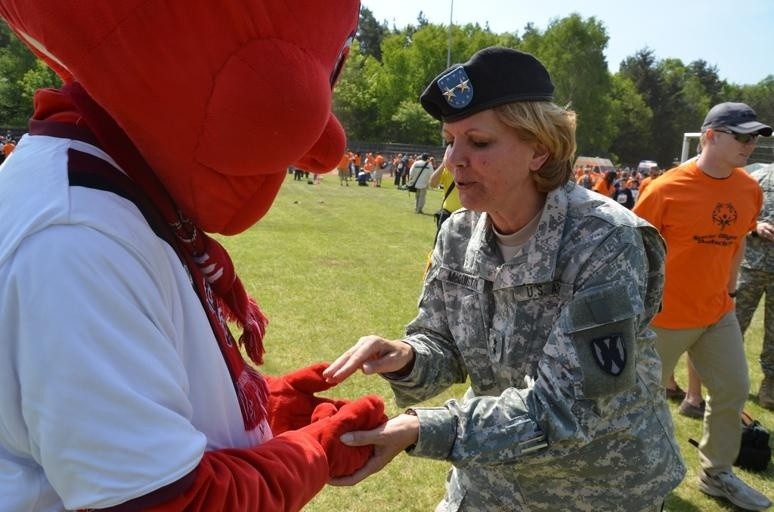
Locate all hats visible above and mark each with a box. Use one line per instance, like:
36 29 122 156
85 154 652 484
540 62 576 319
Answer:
420 47 554 124
702 103 773 137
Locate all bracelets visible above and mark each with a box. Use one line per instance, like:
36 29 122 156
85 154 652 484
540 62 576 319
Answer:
728 290 737 297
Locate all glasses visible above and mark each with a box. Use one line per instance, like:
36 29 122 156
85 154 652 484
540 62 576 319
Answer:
715 130 762 143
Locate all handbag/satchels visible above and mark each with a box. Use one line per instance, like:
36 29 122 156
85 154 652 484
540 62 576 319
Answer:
734 419 774 470
406 182 416 192
435 207 451 230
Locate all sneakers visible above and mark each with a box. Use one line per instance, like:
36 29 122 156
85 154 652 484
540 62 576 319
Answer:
698 467 772 511
665 382 705 419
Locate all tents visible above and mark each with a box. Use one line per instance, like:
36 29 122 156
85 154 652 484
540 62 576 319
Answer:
574 157 617 174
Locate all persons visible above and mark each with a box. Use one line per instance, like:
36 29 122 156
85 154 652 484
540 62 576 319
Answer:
293 169 322 185
0 130 16 166
666 355 706 419
734 162 774 412
322 49 686 512
430 161 463 248
337 153 386 188
390 152 438 213
630 102 772 511
575 167 672 210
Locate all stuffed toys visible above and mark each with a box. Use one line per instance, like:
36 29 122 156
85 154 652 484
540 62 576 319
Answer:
0 0 387 512
